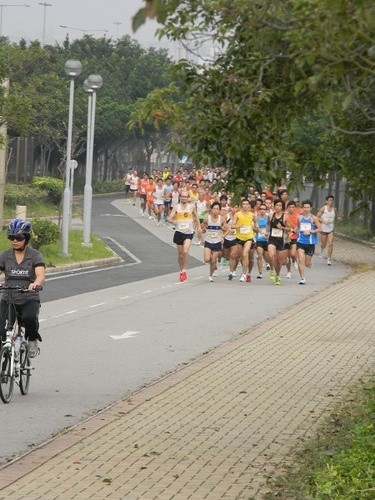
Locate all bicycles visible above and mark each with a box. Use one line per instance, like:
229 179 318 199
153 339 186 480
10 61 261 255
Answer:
0 285 43 403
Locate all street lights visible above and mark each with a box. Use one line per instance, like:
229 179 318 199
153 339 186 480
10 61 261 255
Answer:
0 4 30 37
56 59 82 258
83 75 104 247
113 22 123 40
58 24 108 49
37 3 52 47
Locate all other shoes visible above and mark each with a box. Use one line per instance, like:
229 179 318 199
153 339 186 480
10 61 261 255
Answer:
232 271 236 276
269 273 274 279
214 269 218 277
179 271 187 282
28 339 38 358
274 278 281 285
208 276 214 282
266 264 270 271
227 274 233 280
298 279 306 284
285 272 291 278
327 258 332 265
256 272 263 279
246 274 251 282
240 274 246 281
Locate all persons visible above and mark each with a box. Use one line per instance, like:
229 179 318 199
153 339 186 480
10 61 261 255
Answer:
201 178 322 285
123 163 236 245
167 190 202 282
315 195 338 266
0 219 46 358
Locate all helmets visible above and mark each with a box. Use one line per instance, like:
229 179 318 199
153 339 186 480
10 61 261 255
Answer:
7 219 32 235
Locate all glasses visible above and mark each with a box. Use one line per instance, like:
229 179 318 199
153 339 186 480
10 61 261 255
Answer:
8 234 25 241
260 208 266 210
